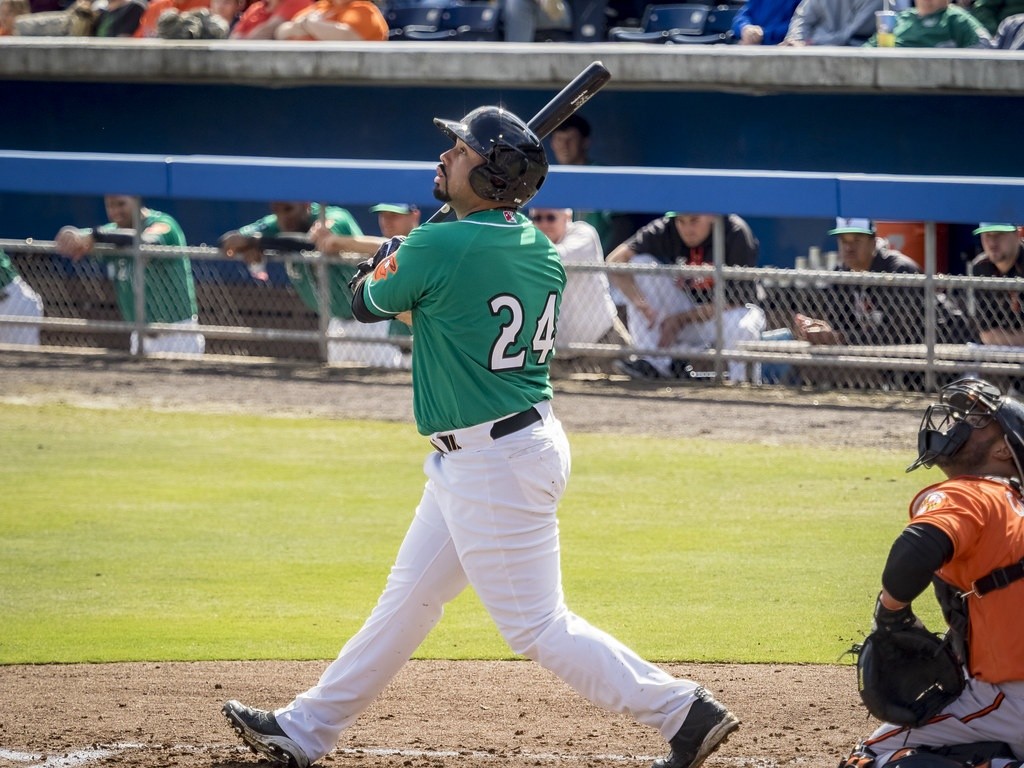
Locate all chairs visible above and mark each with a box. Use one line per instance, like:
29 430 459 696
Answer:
381 0 743 45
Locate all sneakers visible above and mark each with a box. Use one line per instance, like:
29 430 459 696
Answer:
651 686 741 768
221 699 308 768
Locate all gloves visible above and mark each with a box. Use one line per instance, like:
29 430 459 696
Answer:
871 588 926 633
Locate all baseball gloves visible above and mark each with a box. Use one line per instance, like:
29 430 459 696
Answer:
837 589 969 753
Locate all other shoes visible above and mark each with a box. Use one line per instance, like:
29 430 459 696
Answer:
612 357 658 379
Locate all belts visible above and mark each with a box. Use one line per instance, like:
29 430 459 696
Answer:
429 407 542 455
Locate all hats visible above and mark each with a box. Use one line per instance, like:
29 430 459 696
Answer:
827 216 874 236
369 203 413 214
973 222 1015 235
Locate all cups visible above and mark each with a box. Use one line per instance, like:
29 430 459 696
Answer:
875 11 896 47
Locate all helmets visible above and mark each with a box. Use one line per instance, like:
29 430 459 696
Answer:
433 106 549 207
993 395 1023 484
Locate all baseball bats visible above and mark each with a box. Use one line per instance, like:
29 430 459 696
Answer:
349 58 614 295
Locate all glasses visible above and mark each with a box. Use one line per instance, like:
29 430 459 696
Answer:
531 213 556 222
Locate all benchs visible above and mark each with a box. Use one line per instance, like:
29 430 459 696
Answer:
21 276 324 359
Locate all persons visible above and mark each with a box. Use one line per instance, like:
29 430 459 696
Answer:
550 114 612 256
791 219 1024 397
221 200 635 375
52 195 205 354
0 249 43 345
837 376 1024 768
220 104 744 768
605 212 766 386
0 0 1024 50
